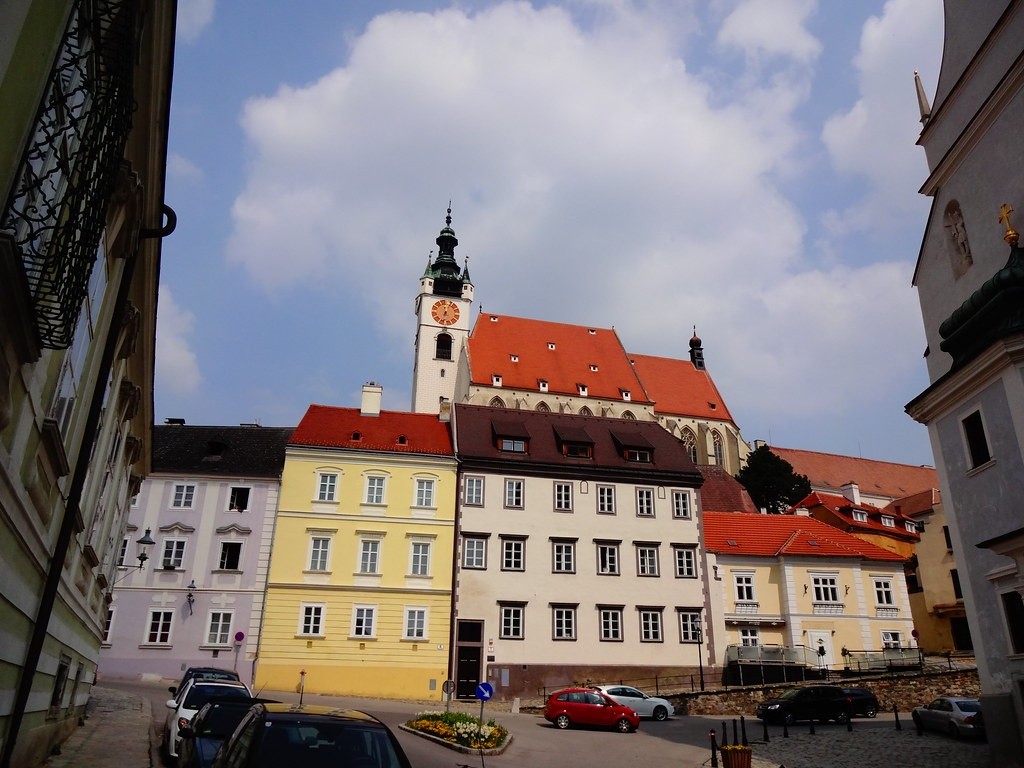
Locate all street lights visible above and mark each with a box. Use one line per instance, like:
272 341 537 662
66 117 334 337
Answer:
694 617 706 691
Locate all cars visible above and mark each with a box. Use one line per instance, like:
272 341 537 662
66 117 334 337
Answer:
593 685 677 721
164 680 256 760
543 686 641 733
210 704 414 768
168 667 239 698
174 697 285 768
843 688 880 718
911 695 984 735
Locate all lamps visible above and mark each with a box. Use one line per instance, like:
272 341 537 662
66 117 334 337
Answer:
186 580 196 615
732 621 738 625
804 584 808 593
771 622 777 625
116 169 148 210
845 584 850 595
113 527 156 586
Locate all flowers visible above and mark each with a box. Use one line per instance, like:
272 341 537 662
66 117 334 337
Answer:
723 744 751 750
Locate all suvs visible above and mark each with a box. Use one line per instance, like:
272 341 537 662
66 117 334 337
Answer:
756 684 854 726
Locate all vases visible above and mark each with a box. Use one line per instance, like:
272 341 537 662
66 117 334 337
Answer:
720 747 752 768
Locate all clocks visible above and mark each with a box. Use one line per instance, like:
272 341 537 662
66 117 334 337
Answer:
431 299 460 325
